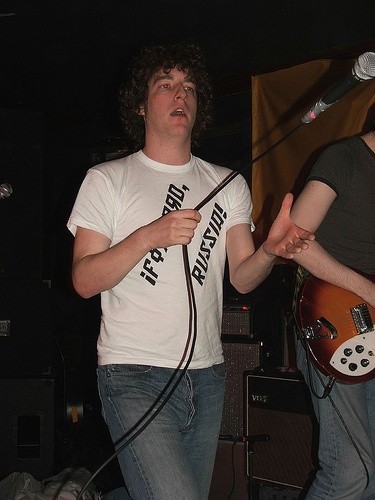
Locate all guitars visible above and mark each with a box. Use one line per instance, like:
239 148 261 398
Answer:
295 275 375 384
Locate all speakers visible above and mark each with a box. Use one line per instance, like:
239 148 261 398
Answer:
243 371 321 491
0 367 64 485
207 292 287 500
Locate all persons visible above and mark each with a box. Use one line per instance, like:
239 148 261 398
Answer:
67 46 316 500
291 103 375 500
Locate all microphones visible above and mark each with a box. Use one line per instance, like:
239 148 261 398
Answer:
301 51 375 124
